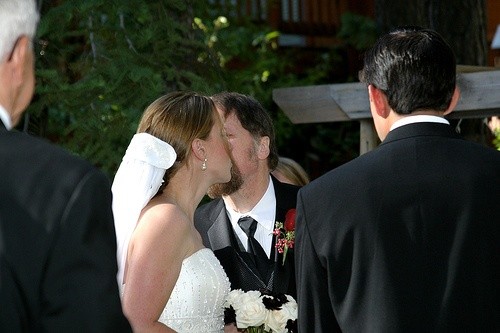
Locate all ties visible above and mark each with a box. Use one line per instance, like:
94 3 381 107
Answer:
237 216 271 283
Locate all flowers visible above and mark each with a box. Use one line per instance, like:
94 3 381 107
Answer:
225 288 298 333
273 209 296 265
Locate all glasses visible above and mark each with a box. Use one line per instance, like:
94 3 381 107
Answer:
6 34 50 64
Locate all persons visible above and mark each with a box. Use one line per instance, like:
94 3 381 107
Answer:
296 29 499 333
111 92 233 333
194 92 310 333
0 0 132 333
